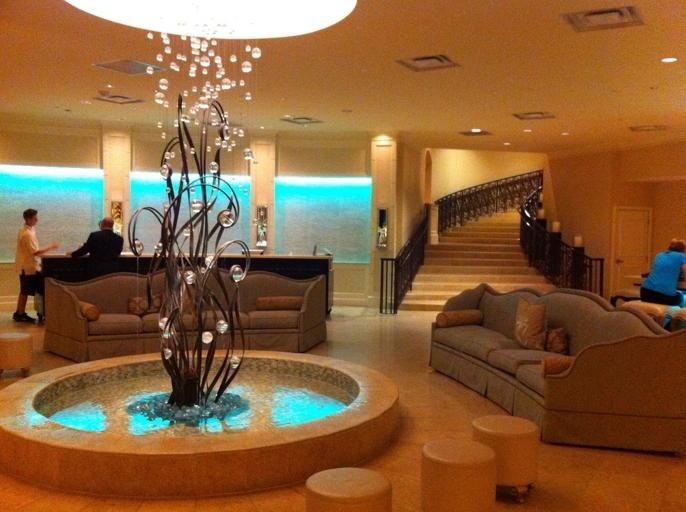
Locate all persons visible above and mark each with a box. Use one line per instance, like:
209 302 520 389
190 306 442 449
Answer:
641 238 686 305
66 218 123 276
13 209 60 322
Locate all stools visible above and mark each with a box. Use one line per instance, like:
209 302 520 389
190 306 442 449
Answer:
667 306 686 332
0 332 33 376
422 438 496 512
624 298 679 329
307 467 392 512
470 415 541 502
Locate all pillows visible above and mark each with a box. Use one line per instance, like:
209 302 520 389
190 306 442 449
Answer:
540 356 574 377
129 293 161 313
516 297 548 350
548 327 567 355
77 299 100 320
254 295 303 309
435 308 482 328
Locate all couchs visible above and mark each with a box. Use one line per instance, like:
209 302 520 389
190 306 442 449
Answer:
44 265 326 364
429 284 686 453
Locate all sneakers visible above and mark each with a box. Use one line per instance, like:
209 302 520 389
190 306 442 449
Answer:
13 312 36 322
